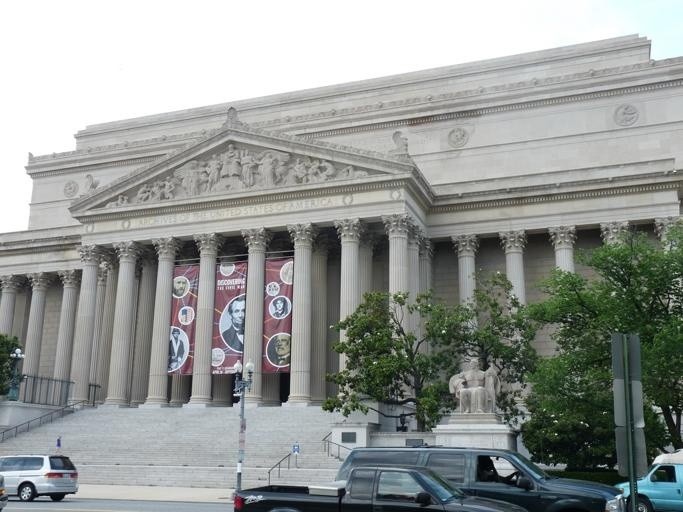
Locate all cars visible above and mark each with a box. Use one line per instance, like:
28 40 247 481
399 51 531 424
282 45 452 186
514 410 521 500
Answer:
333 446 625 511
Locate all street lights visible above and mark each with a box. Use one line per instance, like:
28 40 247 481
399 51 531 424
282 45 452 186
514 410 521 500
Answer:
233 358 254 491
8 347 25 401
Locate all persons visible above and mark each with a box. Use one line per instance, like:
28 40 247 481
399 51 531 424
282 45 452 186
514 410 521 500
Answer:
55 435 62 456
173 275 187 296
273 334 290 366
168 328 184 370
222 295 245 351
452 359 496 413
273 297 288 318
117 144 369 207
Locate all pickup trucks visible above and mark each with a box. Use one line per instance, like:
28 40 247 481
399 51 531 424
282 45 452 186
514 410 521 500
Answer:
234 464 528 512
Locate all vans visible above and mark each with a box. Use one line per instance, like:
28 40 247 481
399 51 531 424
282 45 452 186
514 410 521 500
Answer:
0 455 79 512
614 448 683 512
